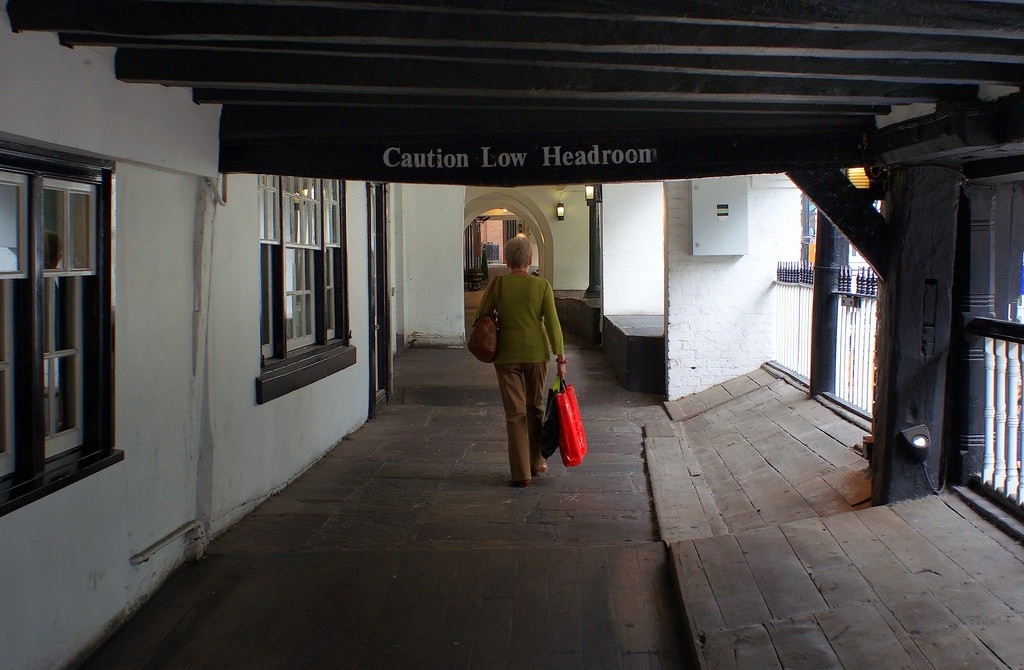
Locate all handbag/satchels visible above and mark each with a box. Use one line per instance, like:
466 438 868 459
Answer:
467 276 499 364
541 376 560 461
555 376 588 467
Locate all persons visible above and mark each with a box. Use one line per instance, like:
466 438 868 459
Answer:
474 236 568 487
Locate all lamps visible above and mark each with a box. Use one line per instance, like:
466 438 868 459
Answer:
901 423 932 466
585 185 595 206
518 223 523 231
557 203 564 221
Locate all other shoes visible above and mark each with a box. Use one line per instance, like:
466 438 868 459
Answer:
531 466 547 476
508 479 531 487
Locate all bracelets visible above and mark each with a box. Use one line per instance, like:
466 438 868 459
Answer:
556 358 568 363
